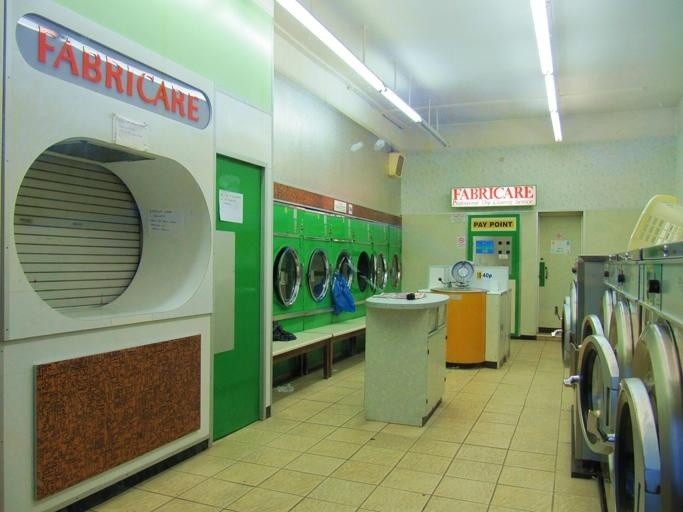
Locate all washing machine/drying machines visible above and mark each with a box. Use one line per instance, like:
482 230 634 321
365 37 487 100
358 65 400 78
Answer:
301 211 329 329
373 219 387 301
273 201 305 337
560 246 681 512
330 214 355 323
352 215 374 320
389 226 403 294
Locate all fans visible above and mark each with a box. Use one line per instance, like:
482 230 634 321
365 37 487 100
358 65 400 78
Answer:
451 261 474 287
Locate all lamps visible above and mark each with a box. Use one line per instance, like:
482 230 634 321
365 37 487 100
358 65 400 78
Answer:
530 0 554 75
550 112 562 142
381 62 423 122
421 99 448 147
544 74 557 112
276 0 384 91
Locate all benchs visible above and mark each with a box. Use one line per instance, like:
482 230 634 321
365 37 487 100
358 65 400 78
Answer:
303 316 366 377
272 332 332 379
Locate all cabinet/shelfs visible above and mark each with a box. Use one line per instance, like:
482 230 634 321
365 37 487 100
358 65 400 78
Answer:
485 288 512 369
363 294 450 427
